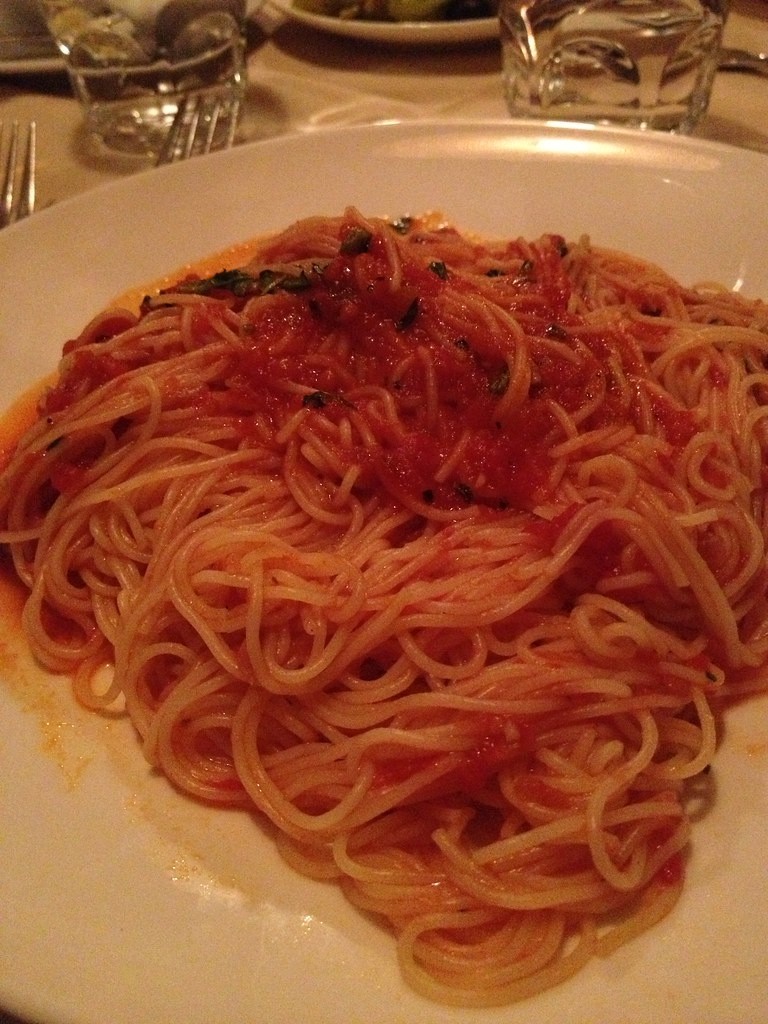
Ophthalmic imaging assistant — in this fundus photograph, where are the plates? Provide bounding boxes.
[280,0,501,45]
[2,119,767,1024]
[0,2,73,72]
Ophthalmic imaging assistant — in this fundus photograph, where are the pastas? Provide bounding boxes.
[0,208,768,1007]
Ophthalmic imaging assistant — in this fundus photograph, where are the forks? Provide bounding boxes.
[1,117,37,227]
[156,92,239,169]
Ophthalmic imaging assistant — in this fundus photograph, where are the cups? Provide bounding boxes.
[501,1,731,135]
[35,0,245,160]
